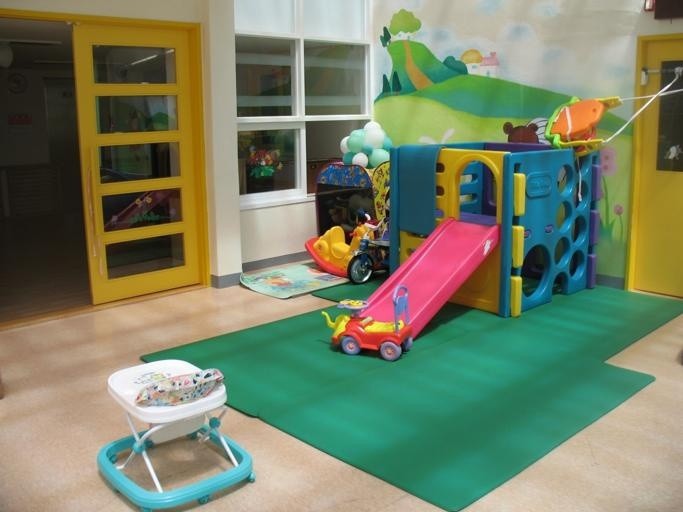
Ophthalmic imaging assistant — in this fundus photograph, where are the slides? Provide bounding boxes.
[353,215,500,343]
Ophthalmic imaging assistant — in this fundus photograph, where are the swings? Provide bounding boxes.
[547,66,683,161]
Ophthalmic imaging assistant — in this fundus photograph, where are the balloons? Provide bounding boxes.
[340,121,393,169]
